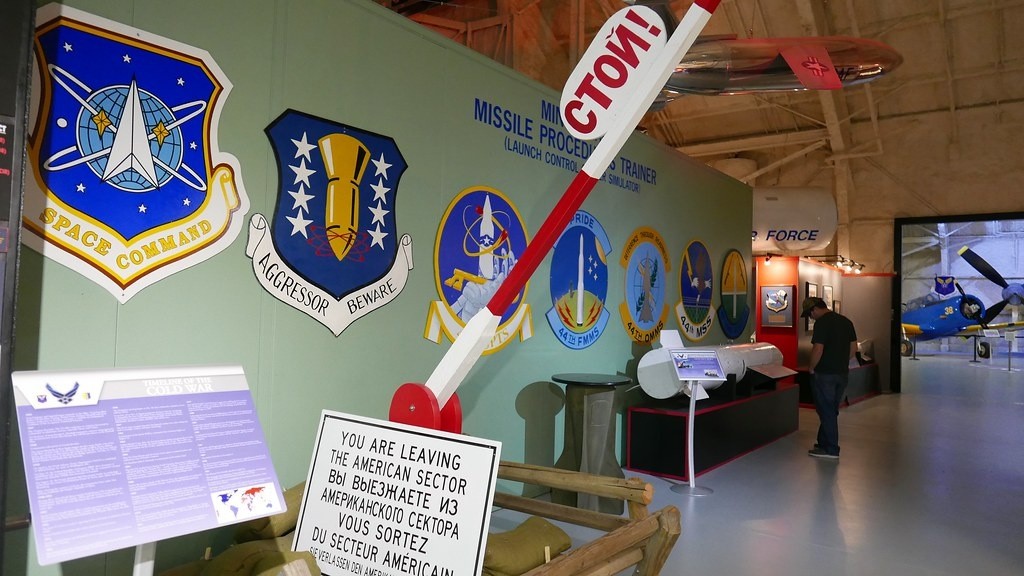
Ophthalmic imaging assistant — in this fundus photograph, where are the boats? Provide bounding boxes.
[651,34,905,110]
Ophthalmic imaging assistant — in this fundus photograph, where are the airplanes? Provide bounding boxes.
[900,280,994,359]
[678,360,692,368]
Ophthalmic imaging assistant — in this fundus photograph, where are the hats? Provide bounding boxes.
[800,297,823,318]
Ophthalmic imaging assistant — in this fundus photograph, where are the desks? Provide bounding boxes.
[551,374,633,515]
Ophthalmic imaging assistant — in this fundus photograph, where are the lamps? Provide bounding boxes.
[764,252,783,265]
[856,264,865,274]
[817,259,855,271]
[804,255,844,266]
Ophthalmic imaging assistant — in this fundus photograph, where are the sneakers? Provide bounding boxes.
[808,444,839,458]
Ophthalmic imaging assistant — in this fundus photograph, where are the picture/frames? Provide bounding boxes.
[823,285,833,311]
[834,300,841,313]
[759,284,796,328]
[805,281,818,331]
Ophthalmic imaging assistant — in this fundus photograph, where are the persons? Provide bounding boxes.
[799,296,859,459]
